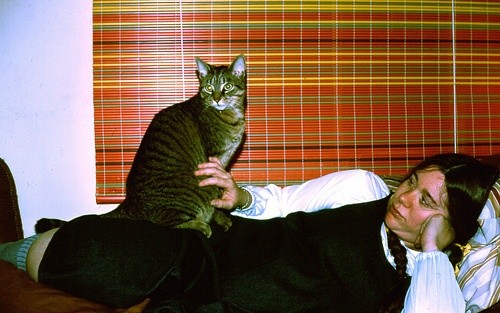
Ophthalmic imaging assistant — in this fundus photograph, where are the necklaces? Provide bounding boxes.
[237,188,250,210]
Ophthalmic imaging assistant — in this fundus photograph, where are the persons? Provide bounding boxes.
[0,152,500,313]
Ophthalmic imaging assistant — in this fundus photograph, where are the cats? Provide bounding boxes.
[35,53,248,239]
